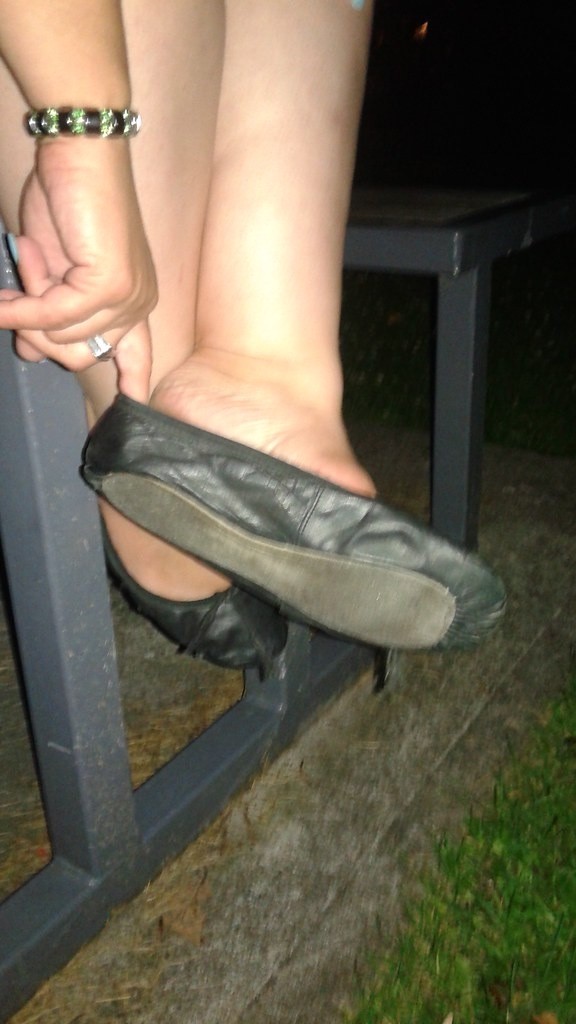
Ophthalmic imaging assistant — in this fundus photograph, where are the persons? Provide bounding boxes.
[0,3,511,671]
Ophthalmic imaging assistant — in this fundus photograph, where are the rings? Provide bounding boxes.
[88,337,117,362]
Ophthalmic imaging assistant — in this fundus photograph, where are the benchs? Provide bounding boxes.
[344,178,575,560]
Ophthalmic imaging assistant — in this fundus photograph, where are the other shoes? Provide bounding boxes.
[98,501,291,670]
[78,391,509,655]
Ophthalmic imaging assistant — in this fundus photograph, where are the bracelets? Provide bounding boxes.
[25,106,143,138]
